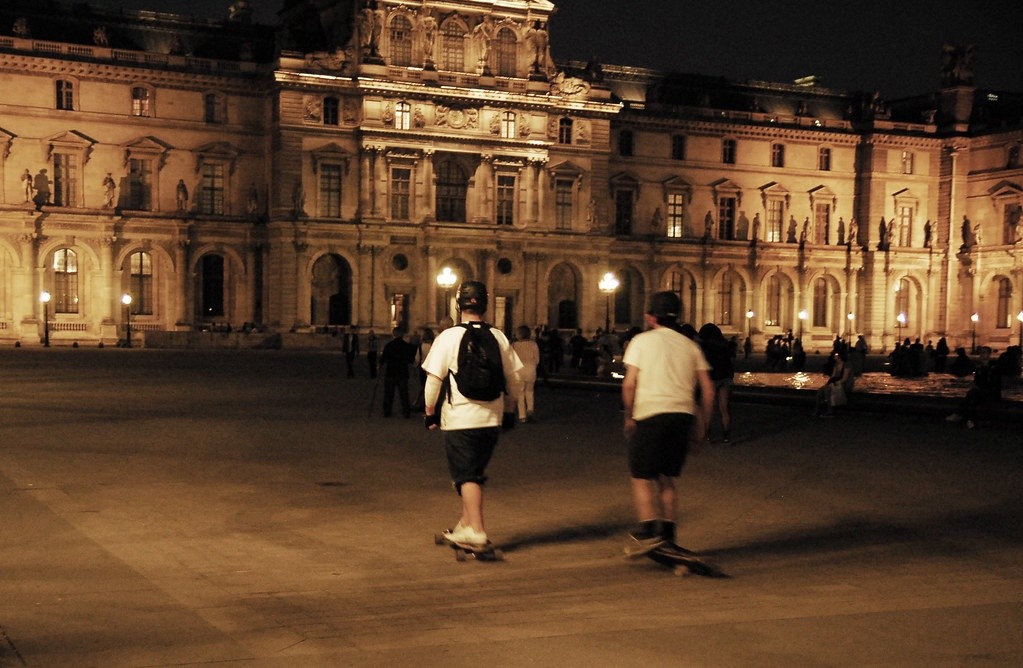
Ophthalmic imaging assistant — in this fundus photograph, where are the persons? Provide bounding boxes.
[972,222,982,245]
[817,333,869,419]
[477,15,491,59]
[885,216,895,239]
[801,216,811,240]
[20,168,33,202]
[419,279,525,545]
[766,335,808,370]
[373,3,385,50]
[421,15,439,63]
[651,207,662,231]
[927,220,936,243]
[176,179,189,210]
[378,326,438,420]
[889,332,1002,428]
[704,210,714,239]
[198,321,341,337]
[753,213,761,240]
[848,217,859,244]
[619,292,754,549]
[247,182,258,216]
[509,325,618,426]
[103,173,116,208]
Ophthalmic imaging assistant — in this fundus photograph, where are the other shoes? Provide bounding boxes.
[445,526,486,547]
[946,413,963,421]
[627,535,676,560]
[966,420,975,429]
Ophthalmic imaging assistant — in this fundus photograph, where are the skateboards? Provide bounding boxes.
[628,529,708,577]
[433,526,506,562]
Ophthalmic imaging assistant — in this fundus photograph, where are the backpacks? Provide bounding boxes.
[450,321,507,402]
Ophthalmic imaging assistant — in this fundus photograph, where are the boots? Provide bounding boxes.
[723,430,730,443]
[705,428,711,443]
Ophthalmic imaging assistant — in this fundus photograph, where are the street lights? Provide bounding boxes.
[897,315,905,345]
[746,310,753,341]
[971,313,978,352]
[598,270,621,335]
[1017,311,1023,347]
[42,293,49,347]
[799,312,806,341]
[847,311,854,345]
[123,295,132,347]
[437,268,456,321]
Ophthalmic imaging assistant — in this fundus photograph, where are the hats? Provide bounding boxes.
[461,281,488,306]
